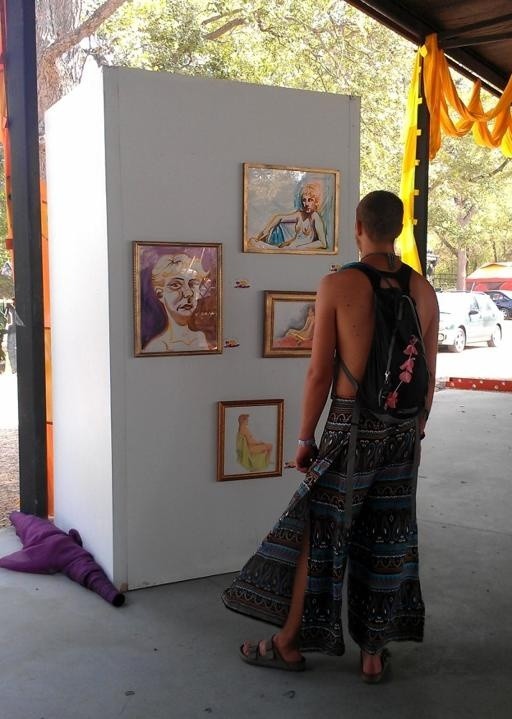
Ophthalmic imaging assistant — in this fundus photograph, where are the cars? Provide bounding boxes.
[435,288,505,354]
[483,288,512,320]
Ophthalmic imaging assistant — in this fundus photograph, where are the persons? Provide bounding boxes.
[254,179,329,250]
[143,252,210,352]
[221,191,440,685]
[285,305,315,341]
[238,413,274,467]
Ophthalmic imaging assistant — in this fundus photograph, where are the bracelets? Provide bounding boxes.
[296,438,317,446]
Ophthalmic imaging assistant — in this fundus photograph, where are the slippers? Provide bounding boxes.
[360,647,391,683]
[237,634,306,671]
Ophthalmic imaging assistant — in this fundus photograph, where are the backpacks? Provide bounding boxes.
[336,263,430,424]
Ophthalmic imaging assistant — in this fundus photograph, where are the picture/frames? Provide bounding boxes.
[243,162,339,255]
[133,240,225,358]
[217,399,286,479]
[264,290,315,358]
